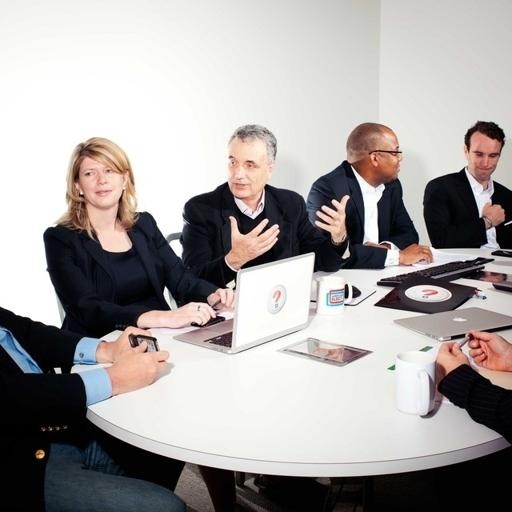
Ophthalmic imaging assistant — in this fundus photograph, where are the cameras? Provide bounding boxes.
[128,333,160,351]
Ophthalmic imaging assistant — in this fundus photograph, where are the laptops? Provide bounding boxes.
[173,250,316,353]
[393,307,512,339]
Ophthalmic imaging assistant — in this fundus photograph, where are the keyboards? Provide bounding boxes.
[378,261,486,284]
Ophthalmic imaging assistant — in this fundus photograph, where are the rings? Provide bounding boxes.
[196,305,202,311]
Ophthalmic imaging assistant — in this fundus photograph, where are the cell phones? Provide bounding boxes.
[190,315,226,327]
[491,250,512,257]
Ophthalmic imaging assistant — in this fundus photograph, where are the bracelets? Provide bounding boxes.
[330,233,349,246]
[481,215,495,229]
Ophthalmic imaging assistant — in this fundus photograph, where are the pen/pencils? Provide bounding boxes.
[458,335,470,349]
[212,300,222,310]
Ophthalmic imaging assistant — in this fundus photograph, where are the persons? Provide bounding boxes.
[45,137,239,370]
[436,330,510,445]
[423,120,511,250]
[305,122,432,271]
[1,308,189,512]
[180,124,353,291]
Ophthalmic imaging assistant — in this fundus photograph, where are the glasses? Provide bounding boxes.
[369,150,403,158]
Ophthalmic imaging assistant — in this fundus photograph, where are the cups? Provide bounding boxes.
[394,351,438,416]
[315,275,354,318]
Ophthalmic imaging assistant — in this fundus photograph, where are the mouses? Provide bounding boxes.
[345,285,362,298]
[473,257,487,264]
